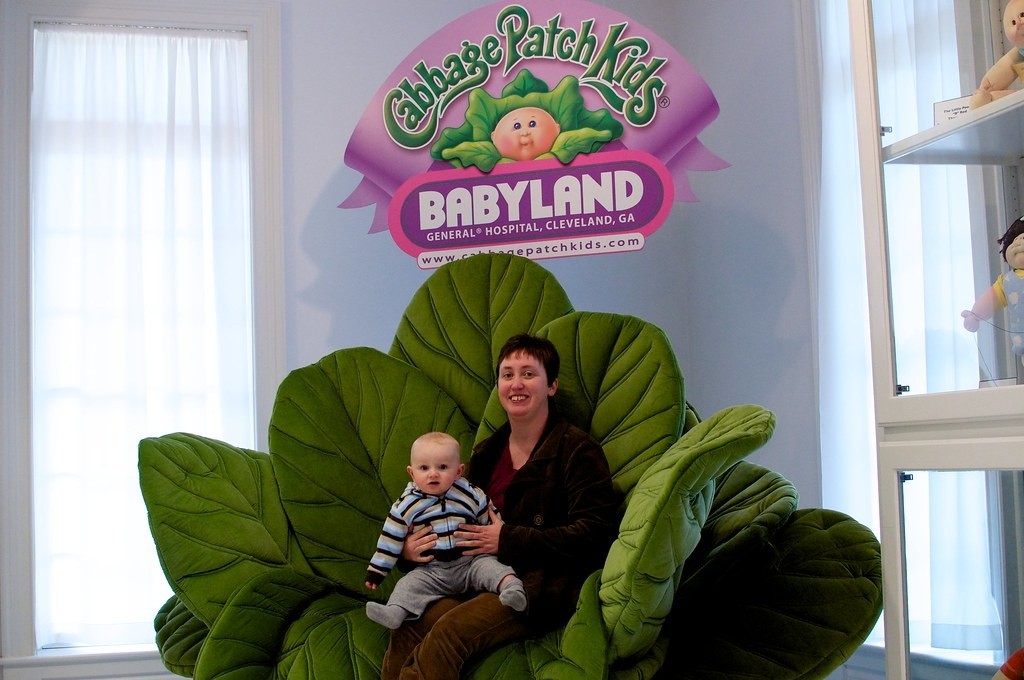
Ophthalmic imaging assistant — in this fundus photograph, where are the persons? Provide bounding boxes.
[381,333,614,680]
[365,432,527,629]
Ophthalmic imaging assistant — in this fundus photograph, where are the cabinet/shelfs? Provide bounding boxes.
[845,0,1024,680]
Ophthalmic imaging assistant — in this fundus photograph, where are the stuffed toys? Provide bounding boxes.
[961,215,1024,366]
[968,0,1024,111]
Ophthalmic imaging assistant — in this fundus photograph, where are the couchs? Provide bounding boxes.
[137,252,882,680]
